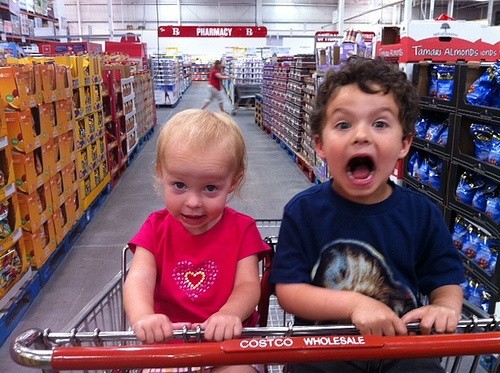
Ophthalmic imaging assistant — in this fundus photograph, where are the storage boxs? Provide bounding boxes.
[0,42,156,346]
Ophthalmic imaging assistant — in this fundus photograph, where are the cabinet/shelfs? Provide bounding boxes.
[0,2,60,42]
[150,53,215,108]
[222,35,500,373]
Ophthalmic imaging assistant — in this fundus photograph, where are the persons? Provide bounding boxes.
[269,54,468,373]
[200,60,235,115]
[124,108,272,373]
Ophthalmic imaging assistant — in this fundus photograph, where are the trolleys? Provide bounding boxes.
[222,74,262,115]
[8,216,499,368]
